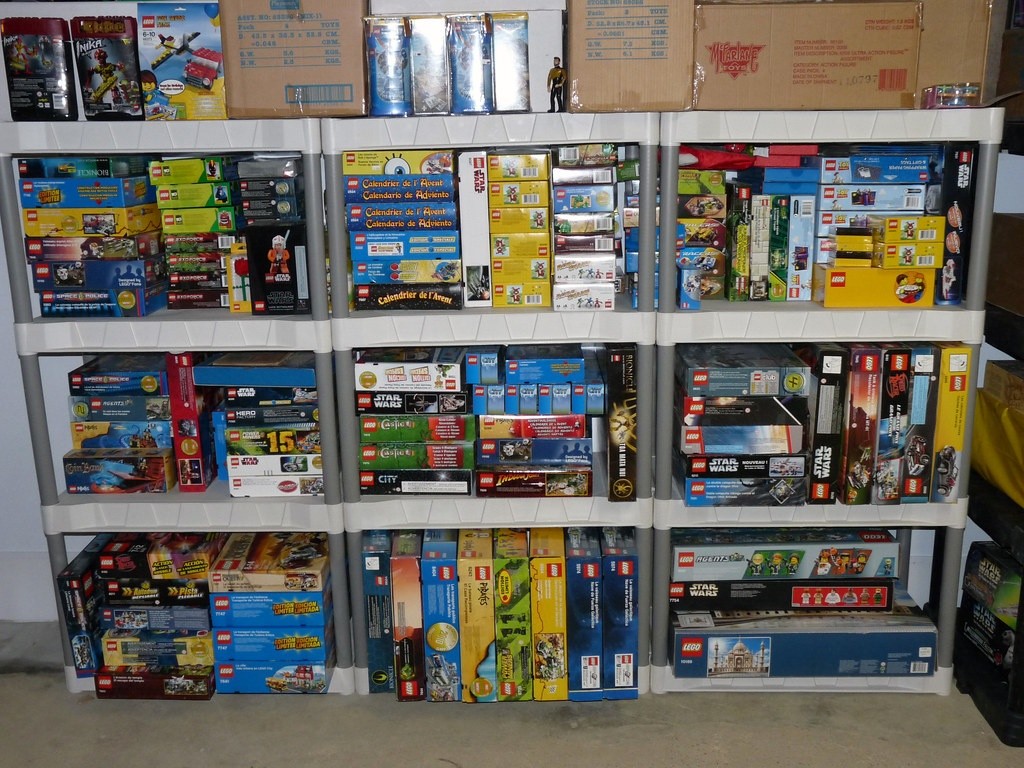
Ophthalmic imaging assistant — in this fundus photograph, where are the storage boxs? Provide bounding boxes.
[0,0,1024,703]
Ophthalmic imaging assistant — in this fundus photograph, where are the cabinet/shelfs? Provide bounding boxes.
[0,117,356,701]
[965,118,1024,580]
[651,106,1005,698]
[320,112,660,696]
